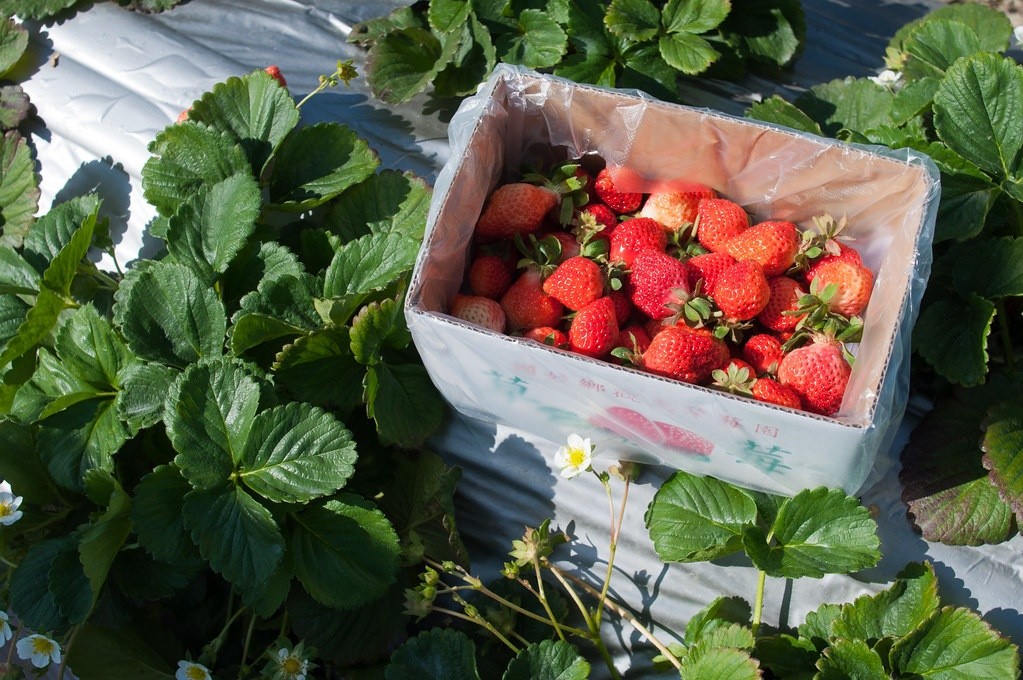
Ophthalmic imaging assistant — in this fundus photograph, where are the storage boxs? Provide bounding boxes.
[403,64,939,501]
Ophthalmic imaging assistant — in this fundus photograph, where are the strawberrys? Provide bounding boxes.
[177,106,192,123]
[265,66,287,88]
[444,164,874,419]
